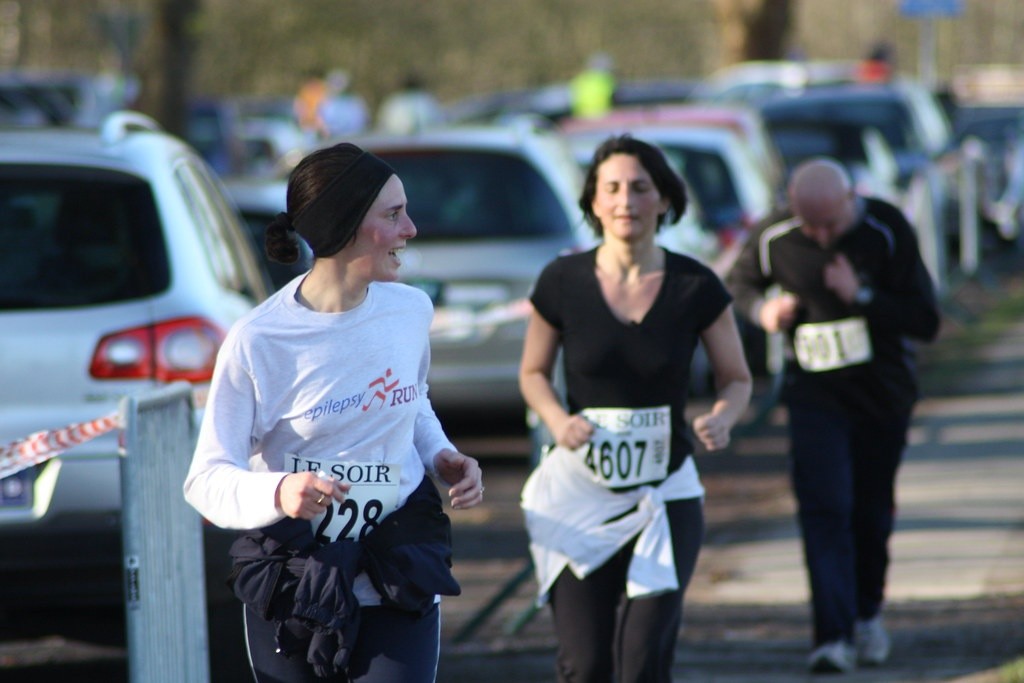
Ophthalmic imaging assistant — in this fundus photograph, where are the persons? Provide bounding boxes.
[293,62,451,140]
[517,132,754,683]
[181,142,486,682]
[723,156,940,673]
[856,36,898,86]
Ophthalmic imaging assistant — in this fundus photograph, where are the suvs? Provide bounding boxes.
[0,108,282,615]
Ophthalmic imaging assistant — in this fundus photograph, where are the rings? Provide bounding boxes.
[480,486,485,494]
[317,493,325,504]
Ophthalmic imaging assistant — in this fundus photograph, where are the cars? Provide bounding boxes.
[196,58,959,434]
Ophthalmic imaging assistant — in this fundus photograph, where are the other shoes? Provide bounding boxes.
[852,613,890,665]
[808,640,856,673]
